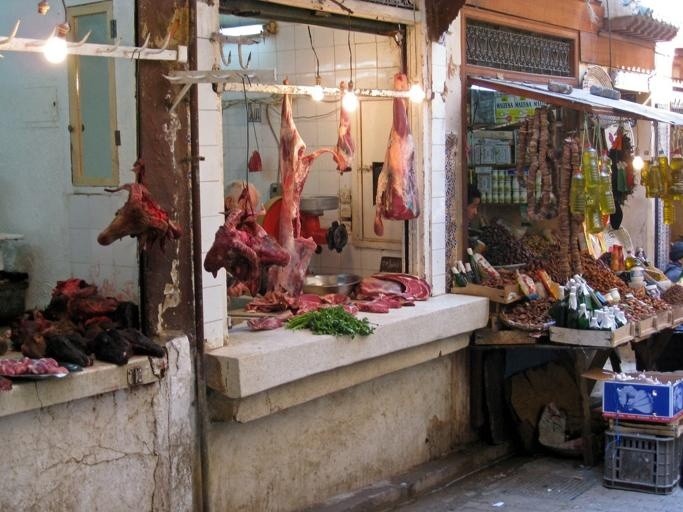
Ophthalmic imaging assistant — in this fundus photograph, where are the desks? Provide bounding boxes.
[471,328,674,465]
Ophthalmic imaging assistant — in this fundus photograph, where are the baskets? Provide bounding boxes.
[603,431,683,494]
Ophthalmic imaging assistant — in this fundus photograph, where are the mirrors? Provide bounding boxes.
[65,1,119,186]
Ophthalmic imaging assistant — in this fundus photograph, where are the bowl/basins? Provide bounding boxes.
[302,273,362,299]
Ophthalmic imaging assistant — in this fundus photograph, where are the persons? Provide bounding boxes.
[224,176,261,299]
[662,241,682,334]
[467,180,489,254]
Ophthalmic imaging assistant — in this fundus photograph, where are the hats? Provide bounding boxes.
[670,241,682,260]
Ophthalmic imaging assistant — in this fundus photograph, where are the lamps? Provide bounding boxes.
[407,2,425,103]
[41,23,69,64]
[632,119,644,172]
[220,24,267,37]
[308,25,323,101]
[343,13,359,112]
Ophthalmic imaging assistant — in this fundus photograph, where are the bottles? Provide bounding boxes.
[554,275,626,331]
[451,248,480,287]
[611,245,635,271]
[569,143,617,233]
[641,149,683,225]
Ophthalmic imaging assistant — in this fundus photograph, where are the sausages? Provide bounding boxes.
[517,106,585,286]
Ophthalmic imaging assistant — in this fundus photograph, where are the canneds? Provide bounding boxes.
[630,263,644,281]
[480,170,543,204]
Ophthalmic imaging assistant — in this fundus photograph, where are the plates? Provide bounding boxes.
[5,370,66,381]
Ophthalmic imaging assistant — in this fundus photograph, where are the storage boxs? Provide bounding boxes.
[602,370,683,418]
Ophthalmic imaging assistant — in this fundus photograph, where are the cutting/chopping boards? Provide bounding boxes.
[226,305,285,326]
